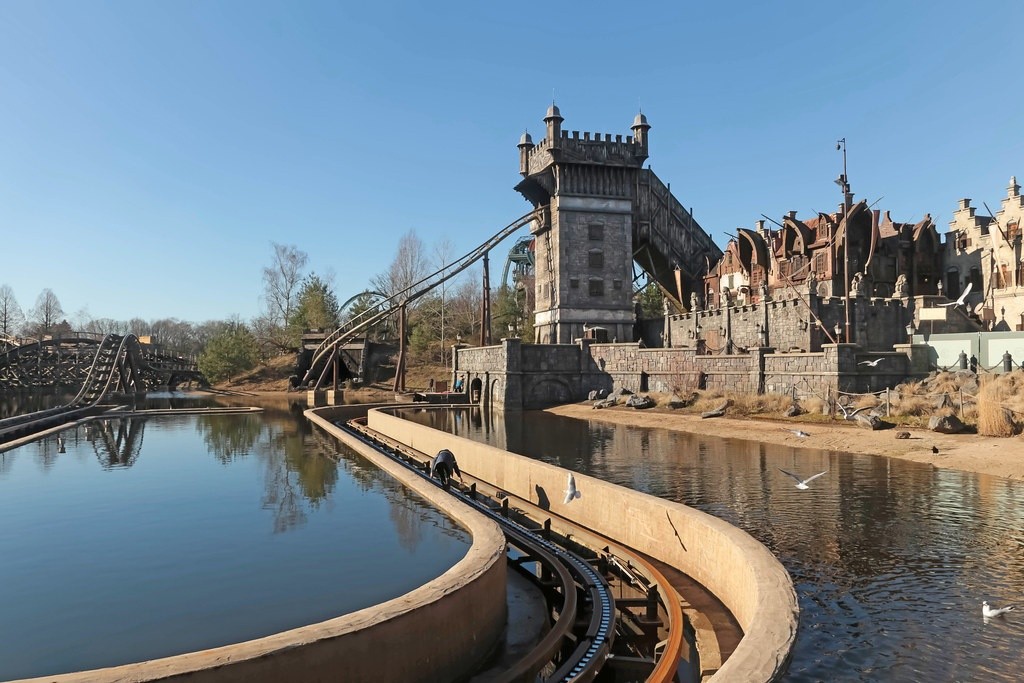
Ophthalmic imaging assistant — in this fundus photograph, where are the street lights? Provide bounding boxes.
[836,137,852,343]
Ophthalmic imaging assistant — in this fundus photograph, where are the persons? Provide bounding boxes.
[454,379,463,393]
[430,449,463,491]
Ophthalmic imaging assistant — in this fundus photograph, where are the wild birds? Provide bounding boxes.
[937,283,973,309]
[931,446,939,455]
[858,357,885,367]
[790,430,810,437]
[982,601,1015,618]
[778,468,829,490]
[836,401,875,420]
[561,472,581,505]
